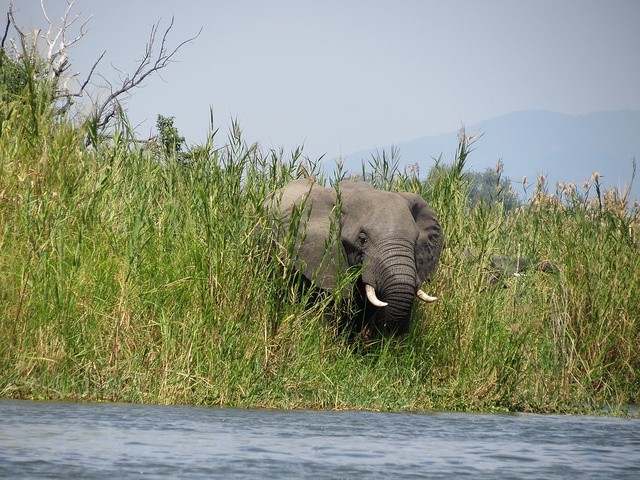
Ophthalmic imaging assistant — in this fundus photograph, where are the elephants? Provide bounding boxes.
[253,177,444,347]
